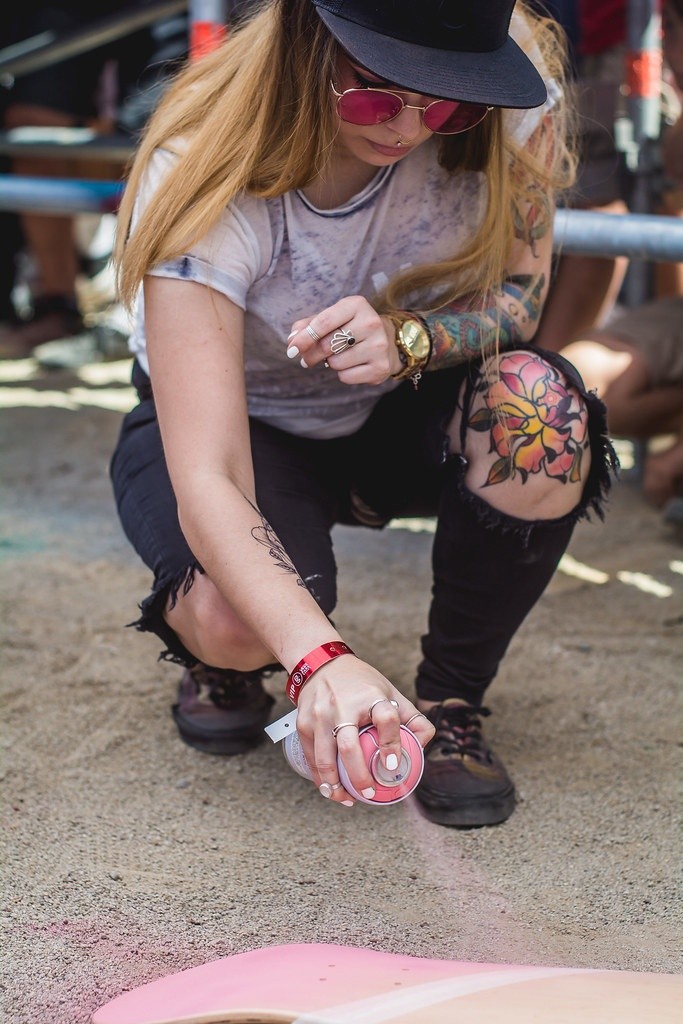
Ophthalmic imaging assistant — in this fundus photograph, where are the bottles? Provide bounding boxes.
[281,723,424,806]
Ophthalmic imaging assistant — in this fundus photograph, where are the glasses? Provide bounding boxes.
[329,76,494,135]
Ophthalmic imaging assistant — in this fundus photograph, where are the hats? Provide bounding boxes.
[312,0,548,109]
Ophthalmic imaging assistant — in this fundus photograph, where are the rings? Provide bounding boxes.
[324,358,330,368]
[404,713,427,727]
[306,325,320,342]
[367,697,399,722]
[332,723,360,738]
[329,328,356,356]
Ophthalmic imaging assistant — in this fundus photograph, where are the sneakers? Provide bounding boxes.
[168,661,275,752]
[415,698,516,825]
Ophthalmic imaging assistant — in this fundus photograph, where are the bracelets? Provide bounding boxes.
[263,640,356,744]
[405,307,432,372]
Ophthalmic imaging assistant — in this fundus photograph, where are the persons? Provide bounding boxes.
[110,0,618,827]
[1,0,682,504]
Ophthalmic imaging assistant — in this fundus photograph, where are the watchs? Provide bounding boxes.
[379,312,431,390]
